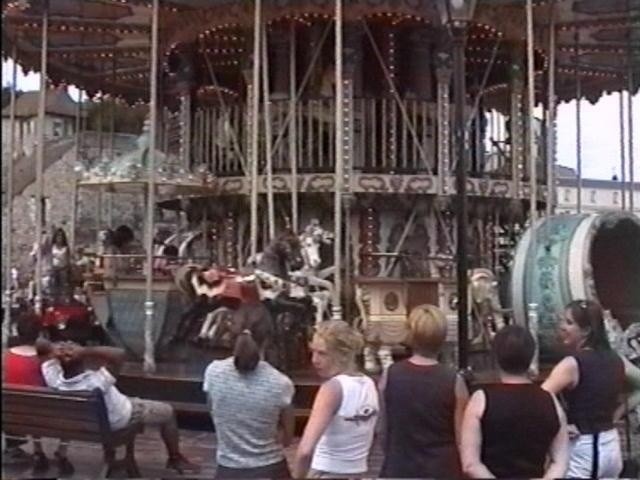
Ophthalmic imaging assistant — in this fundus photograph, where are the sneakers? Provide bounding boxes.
[165,453,200,470]
[2,447,75,473]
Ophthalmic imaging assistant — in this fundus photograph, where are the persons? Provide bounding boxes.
[540,299,639,478]
[459,324,569,480]
[101,226,134,283]
[376,302,470,479]
[35,301,202,475]
[295,318,381,479]
[201,301,295,479]
[497,251,514,327]
[51,229,72,290]
[2,309,79,478]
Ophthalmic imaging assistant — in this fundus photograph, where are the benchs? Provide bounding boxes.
[0,384,147,479]
